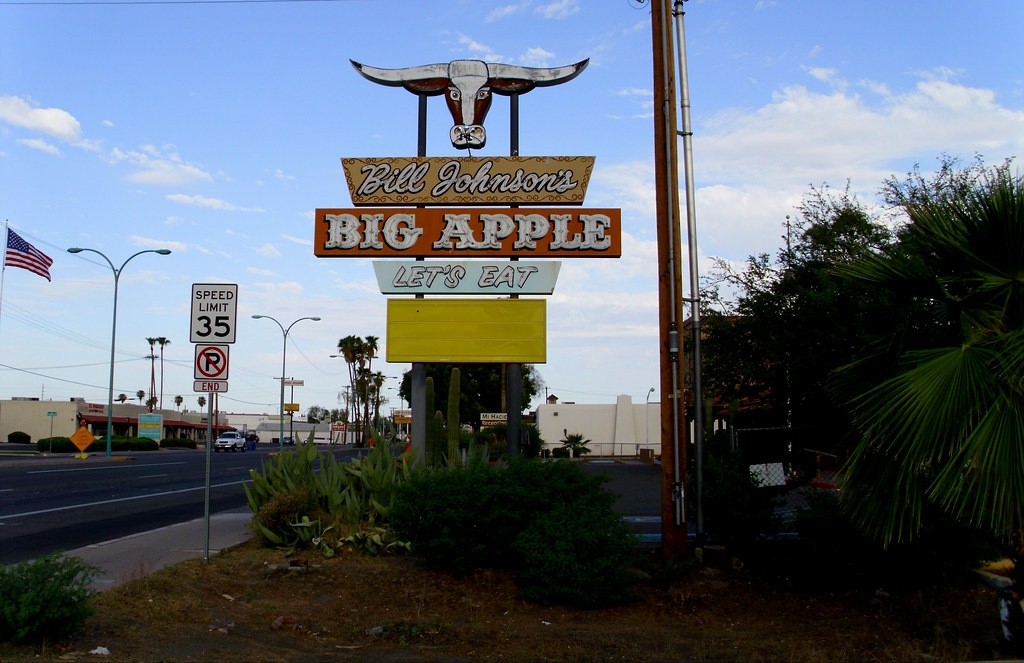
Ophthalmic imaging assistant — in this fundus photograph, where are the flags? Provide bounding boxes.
[5,227,53,282]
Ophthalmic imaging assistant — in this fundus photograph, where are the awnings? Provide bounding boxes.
[82,415,195,427]
[192,425,236,431]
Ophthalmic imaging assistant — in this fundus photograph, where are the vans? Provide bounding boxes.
[304,437,330,445]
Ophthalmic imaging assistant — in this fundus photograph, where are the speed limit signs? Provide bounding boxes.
[190,282,237,345]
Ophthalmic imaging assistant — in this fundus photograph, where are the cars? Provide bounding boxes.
[283,436,294,445]
[215,432,248,453]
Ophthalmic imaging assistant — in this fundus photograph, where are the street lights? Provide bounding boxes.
[330,355,378,449]
[646,387,655,449]
[67,247,173,457]
[387,387,404,439]
[252,314,321,454]
